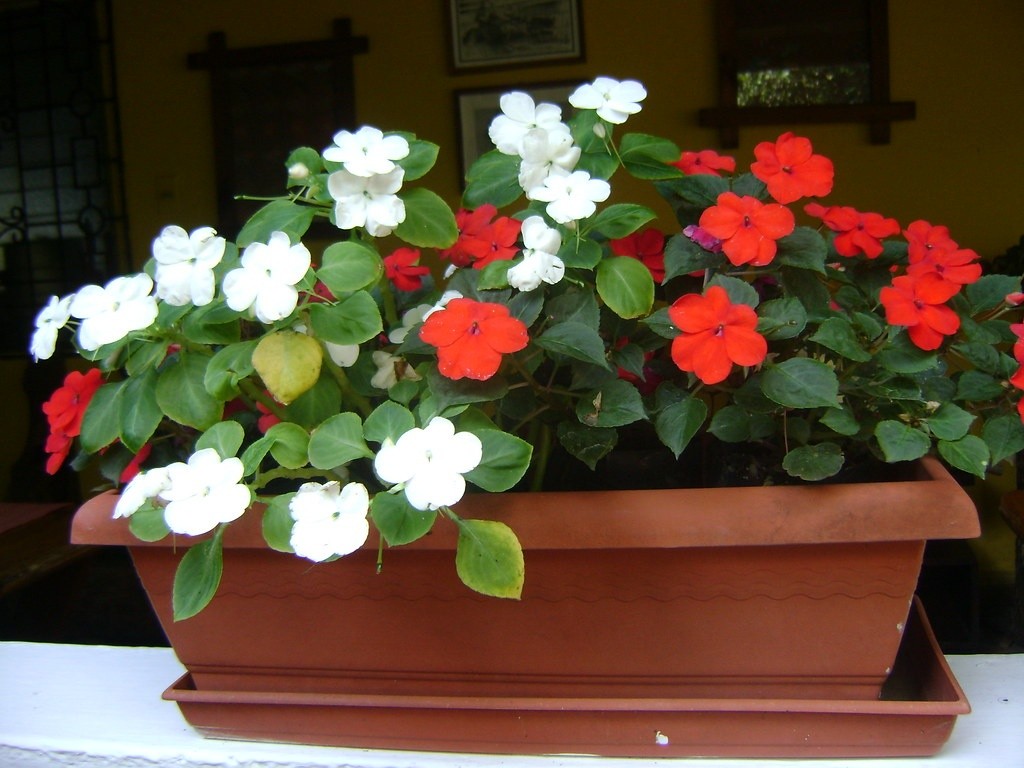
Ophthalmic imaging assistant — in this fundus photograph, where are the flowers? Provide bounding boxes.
[29,78,1024,621]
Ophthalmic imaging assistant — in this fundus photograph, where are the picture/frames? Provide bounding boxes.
[699,0,918,150]
[186,17,371,243]
[452,78,593,197]
[444,0,586,71]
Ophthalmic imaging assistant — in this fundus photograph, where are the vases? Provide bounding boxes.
[71,459,978,699]
